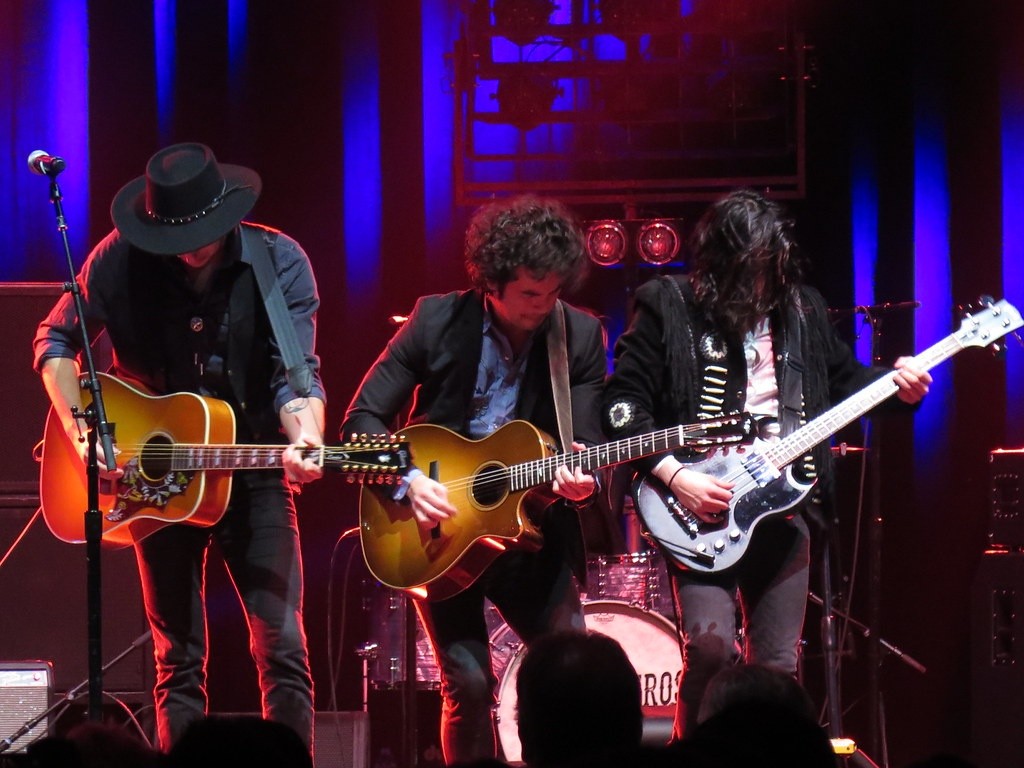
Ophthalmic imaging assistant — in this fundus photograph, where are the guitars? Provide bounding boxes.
[636,294,1024,574]
[359,410,759,604]
[38,371,415,553]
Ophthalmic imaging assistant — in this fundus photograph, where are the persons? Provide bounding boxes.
[34,141,328,755]
[24,629,838,768]
[604,189,934,745]
[339,191,609,768]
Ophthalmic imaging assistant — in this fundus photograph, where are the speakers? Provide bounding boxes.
[207,711,371,768]
[0,660,57,756]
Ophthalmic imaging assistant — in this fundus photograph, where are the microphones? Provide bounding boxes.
[28,150,65,176]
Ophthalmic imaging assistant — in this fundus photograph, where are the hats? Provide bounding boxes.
[110,143,261,255]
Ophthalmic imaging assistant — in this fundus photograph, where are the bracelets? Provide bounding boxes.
[668,466,686,489]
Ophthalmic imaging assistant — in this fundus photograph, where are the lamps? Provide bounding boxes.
[580,218,686,271]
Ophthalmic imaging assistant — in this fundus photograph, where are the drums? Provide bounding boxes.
[466,598,694,768]
[364,571,513,692]
[571,547,679,632]
